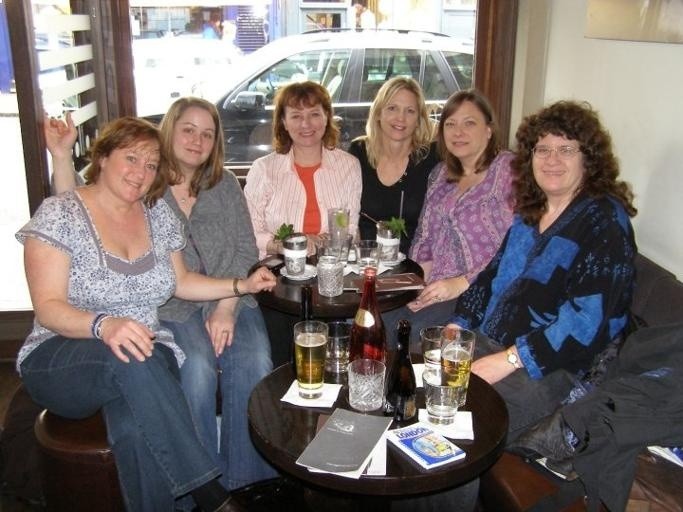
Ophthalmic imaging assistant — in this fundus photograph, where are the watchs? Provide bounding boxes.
[506,349,522,371]
[231,276,243,297]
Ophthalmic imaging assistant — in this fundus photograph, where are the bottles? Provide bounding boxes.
[383,320,416,421]
[349,268,387,376]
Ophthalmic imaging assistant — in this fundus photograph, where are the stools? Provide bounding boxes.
[36,410,113,511]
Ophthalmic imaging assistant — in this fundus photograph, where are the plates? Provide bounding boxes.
[370,251,407,267]
[279,264,316,282]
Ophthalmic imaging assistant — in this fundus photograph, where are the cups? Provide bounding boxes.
[328,207,349,240]
[419,326,444,383]
[348,359,386,411]
[283,234,306,275]
[293,321,329,400]
[325,234,352,268]
[324,322,353,373]
[439,328,476,407]
[423,367,458,425]
[356,241,382,270]
[376,222,400,265]
[316,255,344,297]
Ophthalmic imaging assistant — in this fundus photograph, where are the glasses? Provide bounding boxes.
[530,144,581,159]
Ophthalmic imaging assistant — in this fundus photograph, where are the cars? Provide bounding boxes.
[137,30,474,165]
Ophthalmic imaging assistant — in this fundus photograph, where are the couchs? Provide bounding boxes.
[486,252,683,510]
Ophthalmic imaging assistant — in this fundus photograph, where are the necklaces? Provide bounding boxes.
[172,184,193,204]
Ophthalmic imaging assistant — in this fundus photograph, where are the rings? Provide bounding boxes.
[221,329,228,334]
[432,295,444,302]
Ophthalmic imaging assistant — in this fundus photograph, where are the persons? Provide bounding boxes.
[38,94,294,511]
[383,89,521,340]
[16,118,244,512]
[390,99,639,511]
[245,81,364,256]
[348,77,446,251]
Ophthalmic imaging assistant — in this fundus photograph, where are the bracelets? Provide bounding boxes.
[90,313,113,341]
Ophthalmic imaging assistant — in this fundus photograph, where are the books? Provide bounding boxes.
[354,273,425,293]
[292,408,394,481]
[386,420,463,472]
[342,278,358,290]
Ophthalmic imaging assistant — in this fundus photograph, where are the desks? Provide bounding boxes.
[247,240,423,340]
[246,348,508,512]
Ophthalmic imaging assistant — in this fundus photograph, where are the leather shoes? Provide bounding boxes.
[188,480,234,508]
